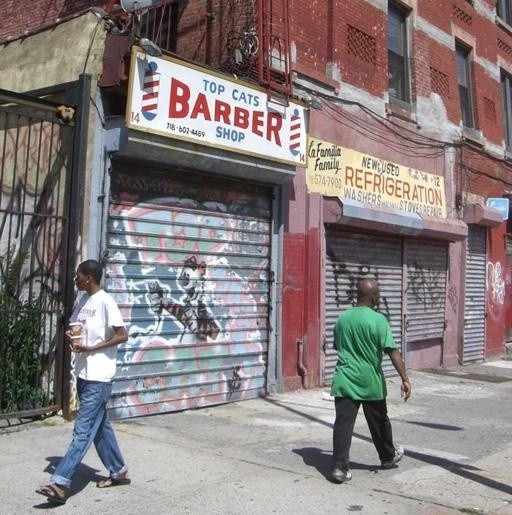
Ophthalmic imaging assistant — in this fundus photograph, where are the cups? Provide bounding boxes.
[68,321,82,336]
[71,336,83,349]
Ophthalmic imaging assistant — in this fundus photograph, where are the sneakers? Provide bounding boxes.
[381,446,405,468]
[332,468,351,482]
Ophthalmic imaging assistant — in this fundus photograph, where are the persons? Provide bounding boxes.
[34,259,131,504]
[329,278,412,483]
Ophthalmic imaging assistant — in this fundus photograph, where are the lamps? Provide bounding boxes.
[139,37,162,57]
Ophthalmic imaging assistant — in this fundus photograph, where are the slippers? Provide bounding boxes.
[97,477,130,488]
[35,484,66,504]
